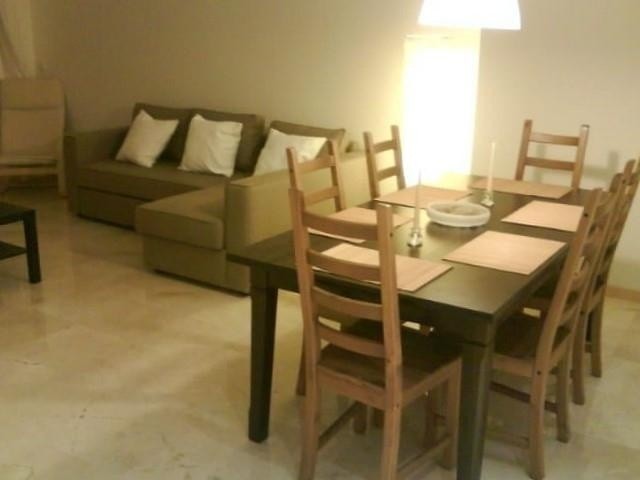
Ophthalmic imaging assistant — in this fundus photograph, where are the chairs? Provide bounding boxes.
[0,78,66,198]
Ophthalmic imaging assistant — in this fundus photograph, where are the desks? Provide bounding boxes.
[225,176,612,480]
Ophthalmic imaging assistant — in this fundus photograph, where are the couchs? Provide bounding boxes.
[67,102,370,295]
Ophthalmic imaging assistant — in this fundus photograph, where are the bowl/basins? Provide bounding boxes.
[426,200,492,227]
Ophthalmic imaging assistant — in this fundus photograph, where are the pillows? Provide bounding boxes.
[114,103,345,178]
